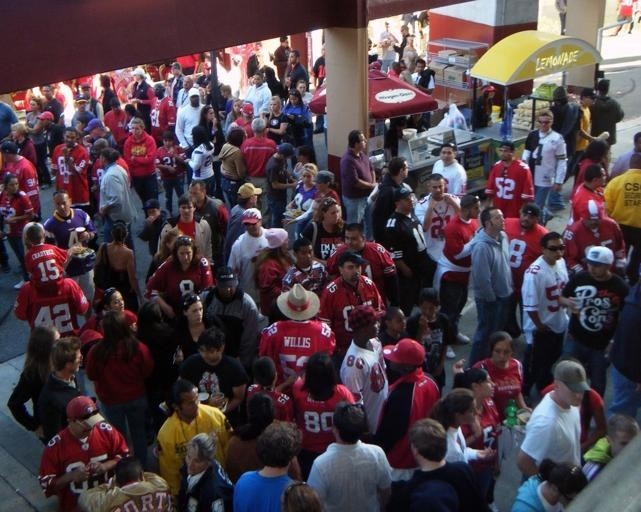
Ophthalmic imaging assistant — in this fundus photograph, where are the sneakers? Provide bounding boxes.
[447,346,456,359]
[168,211,173,218]
[14,280,25,289]
[40,184,51,189]
[457,333,470,343]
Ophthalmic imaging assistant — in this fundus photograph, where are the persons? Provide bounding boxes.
[1,21,641,512]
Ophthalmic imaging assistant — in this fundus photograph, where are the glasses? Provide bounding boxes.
[178,237,192,242]
[289,91,297,94]
[546,244,566,251]
[305,166,313,169]
[538,120,551,123]
[105,287,115,293]
[464,196,479,207]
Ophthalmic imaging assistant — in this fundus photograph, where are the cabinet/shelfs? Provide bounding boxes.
[425,36,490,91]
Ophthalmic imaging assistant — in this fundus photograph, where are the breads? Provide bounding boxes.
[511,99,551,131]
[597,131,609,142]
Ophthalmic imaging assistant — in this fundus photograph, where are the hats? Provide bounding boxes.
[383,339,425,366]
[278,144,299,157]
[540,459,588,494]
[521,203,540,215]
[131,68,144,75]
[265,228,287,248]
[83,119,103,131]
[551,87,568,100]
[500,141,515,150]
[240,103,254,113]
[582,246,614,266]
[555,360,592,392]
[36,111,54,121]
[237,183,262,198]
[216,266,238,286]
[241,208,261,224]
[394,188,414,201]
[66,396,105,427]
[76,95,88,103]
[313,171,333,182]
[0,141,20,154]
[581,88,600,98]
[339,251,369,265]
[277,284,320,320]
[189,88,200,98]
[579,199,603,218]
[142,199,160,209]
[348,306,385,328]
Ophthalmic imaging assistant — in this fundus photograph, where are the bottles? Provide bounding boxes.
[505,398,519,426]
